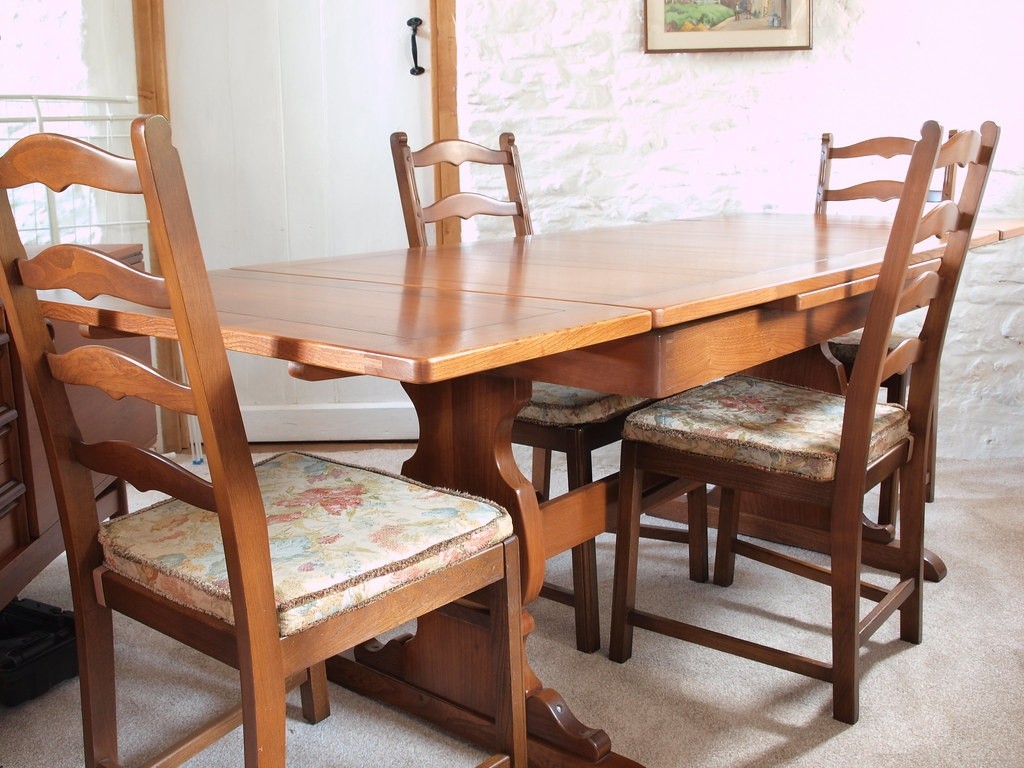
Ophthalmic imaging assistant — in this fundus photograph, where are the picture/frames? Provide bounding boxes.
[643,0,813,54]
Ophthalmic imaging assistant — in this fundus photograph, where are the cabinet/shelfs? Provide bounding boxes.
[0,245,157,609]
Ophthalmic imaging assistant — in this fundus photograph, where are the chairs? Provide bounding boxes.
[603,121,1000,725]
[811,129,963,530]
[390,131,708,653]
[0,114,529,768]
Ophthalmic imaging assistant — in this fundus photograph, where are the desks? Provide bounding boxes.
[38,213,1024,768]
